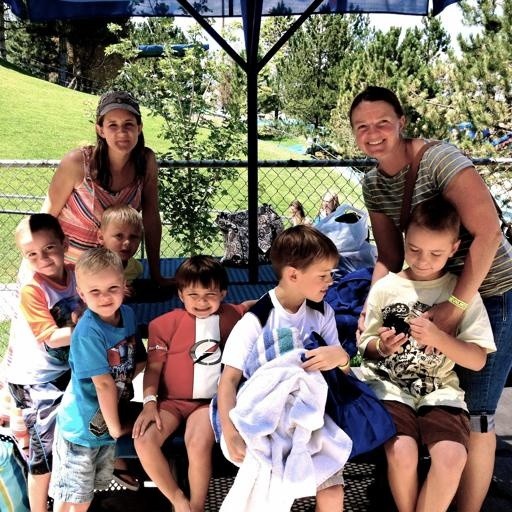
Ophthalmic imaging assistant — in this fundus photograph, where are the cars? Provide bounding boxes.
[237,113,328,143]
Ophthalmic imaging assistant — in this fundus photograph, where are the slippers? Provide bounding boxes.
[113,458,140,492]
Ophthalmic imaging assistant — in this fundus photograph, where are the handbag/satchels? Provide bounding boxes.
[327,266,376,315]
[216,202,284,268]
[315,206,377,281]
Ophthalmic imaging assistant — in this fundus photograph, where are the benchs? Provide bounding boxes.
[113,257,432,502]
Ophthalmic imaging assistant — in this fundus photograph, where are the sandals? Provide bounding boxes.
[12,435,29,458]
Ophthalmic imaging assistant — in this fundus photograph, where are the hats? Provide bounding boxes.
[96,91,141,124]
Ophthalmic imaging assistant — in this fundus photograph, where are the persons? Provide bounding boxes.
[290,201,311,226]
[2,92,162,429]
[313,192,339,225]
[48,246,147,512]
[4,212,141,512]
[94,207,145,301]
[350,203,497,511]
[348,85,512,511]
[129,255,246,512]
[208,226,358,511]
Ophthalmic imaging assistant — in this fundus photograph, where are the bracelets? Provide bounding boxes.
[142,395,157,405]
[339,354,351,368]
[447,294,473,315]
[375,338,390,360]
